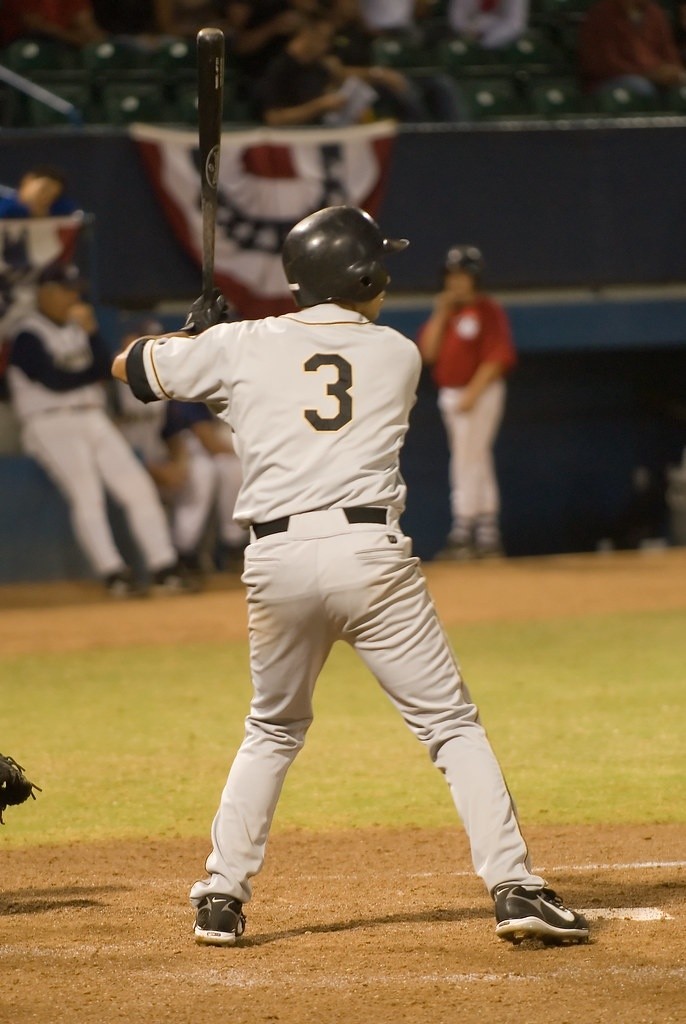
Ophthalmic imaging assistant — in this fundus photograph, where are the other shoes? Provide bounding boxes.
[103,575,149,601]
[471,542,506,559]
[434,541,467,559]
[152,572,201,595]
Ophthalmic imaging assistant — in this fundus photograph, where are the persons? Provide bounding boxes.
[110,205,592,946]
[0,162,249,600]
[0,0,531,131]
[417,245,517,561]
[576,0,686,113]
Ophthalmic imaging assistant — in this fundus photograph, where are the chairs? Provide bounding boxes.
[7,26,686,128]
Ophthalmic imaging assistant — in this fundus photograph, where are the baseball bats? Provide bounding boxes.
[195,27,226,313]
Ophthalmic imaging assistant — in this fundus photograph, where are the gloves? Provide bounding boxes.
[182,287,229,336]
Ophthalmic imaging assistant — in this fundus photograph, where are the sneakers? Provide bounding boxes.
[495,885,589,943]
[193,894,246,944]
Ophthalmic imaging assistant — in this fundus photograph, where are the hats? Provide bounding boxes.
[39,263,91,290]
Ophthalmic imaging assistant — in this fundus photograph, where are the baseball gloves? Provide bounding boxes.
[1,754,42,825]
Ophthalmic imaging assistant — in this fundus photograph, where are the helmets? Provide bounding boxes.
[281,205,410,308]
[444,245,486,276]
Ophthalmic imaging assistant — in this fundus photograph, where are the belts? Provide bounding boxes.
[253,506,387,539]
